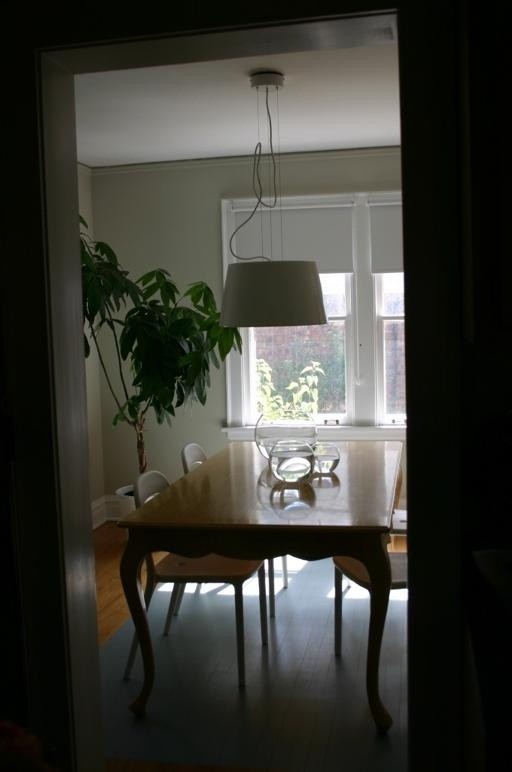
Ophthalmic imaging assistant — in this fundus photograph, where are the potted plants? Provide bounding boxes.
[81,214,242,543]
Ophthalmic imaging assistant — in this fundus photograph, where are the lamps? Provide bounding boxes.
[220,72,328,326]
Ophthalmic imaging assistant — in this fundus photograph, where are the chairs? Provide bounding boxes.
[324,549,408,669]
[122,468,275,702]
[387,443,409,543]
[177,446,293,618]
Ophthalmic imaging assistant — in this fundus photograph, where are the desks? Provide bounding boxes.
[125,435,404,701]
